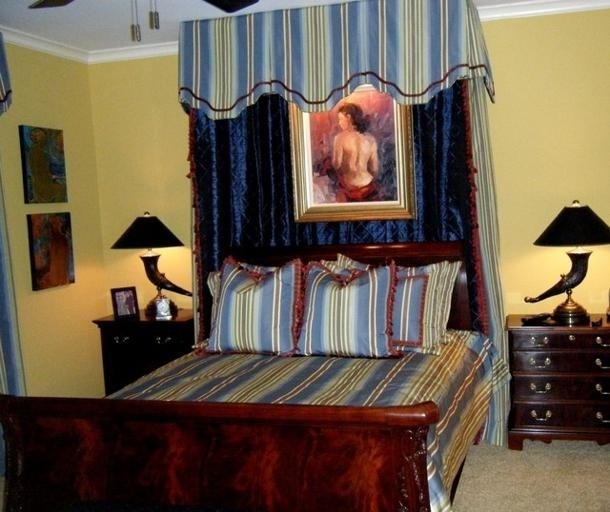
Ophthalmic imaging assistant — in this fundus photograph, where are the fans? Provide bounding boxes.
[28,0,260,13]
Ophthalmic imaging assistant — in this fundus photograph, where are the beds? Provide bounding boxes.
[0,241,514,512]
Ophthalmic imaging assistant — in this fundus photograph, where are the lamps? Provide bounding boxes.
[525,200,610,326]
[111,211,192,317]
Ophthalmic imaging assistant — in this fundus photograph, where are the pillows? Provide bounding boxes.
[192,253,463,358]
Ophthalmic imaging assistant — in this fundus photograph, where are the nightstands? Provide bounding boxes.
[92,308,195,396]
[504,313,610,451]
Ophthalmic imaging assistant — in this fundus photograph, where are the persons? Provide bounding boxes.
[323,103,382,204]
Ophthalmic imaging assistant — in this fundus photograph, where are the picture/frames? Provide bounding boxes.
[111,286,139,320]
[289,84,418,223]
